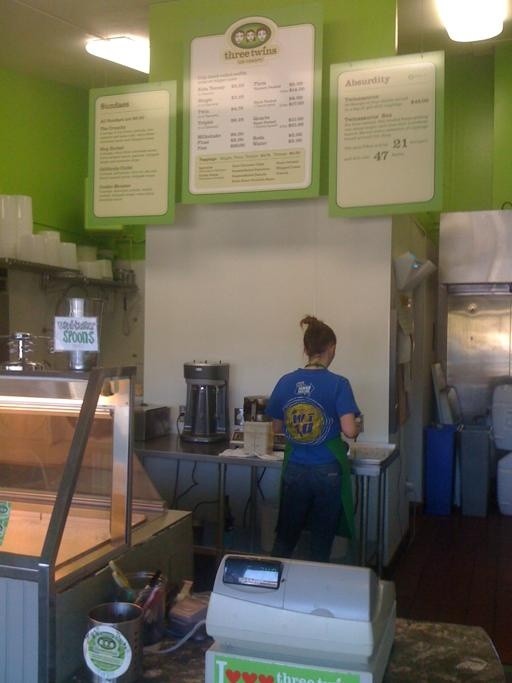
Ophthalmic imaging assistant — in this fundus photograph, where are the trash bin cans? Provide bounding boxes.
[423,422,489,518]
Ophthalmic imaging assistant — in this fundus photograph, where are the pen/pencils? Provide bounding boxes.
[108,560,167,641]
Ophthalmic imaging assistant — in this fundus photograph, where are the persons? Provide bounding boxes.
[263,316,364,561]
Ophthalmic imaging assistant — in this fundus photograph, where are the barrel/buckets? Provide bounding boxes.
[492,384,512,451]
[497,452,512,516]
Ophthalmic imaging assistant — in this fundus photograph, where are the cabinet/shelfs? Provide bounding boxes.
[0,364,193,683]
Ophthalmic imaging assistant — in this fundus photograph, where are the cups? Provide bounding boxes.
[112,571,166,646]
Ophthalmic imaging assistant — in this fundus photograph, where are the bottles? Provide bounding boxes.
[244,396,269,422]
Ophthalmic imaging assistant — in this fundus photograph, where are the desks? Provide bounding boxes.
[64,590,502,682]
[130,436,387,577]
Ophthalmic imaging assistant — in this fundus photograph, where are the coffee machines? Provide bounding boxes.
[180,360,229,443]
